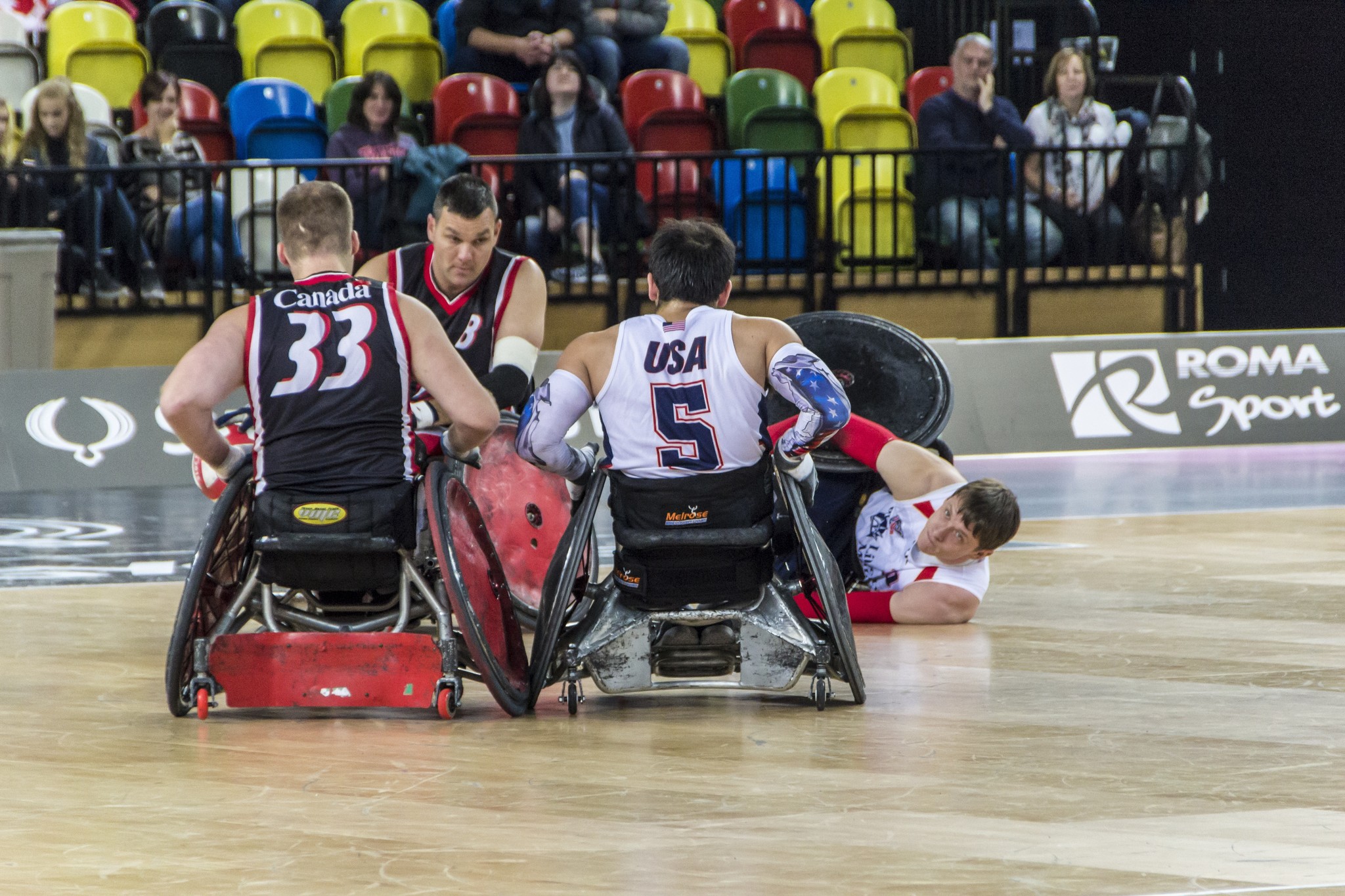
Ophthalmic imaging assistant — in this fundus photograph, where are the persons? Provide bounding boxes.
[0,0,1139,624]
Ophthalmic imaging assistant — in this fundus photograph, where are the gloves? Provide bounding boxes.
[214,443,253,483]
[213,404,253,434]
[565,442,600,516]
[440,430,482,470]
[774,437,819,509]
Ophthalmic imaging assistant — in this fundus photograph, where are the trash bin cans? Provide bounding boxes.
[0,226,64,369]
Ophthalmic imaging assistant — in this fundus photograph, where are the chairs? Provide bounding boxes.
[0,0,955,275]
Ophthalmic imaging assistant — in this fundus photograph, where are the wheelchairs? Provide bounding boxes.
[163,404,536,721]
[415,408,599,633]
[529,425,868,713]
[764,310,956,616]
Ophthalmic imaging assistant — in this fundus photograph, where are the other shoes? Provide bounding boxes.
[227,253,268,289]
[137,264,167,300]
[549,257,609,283]
[80,266,129,298]
